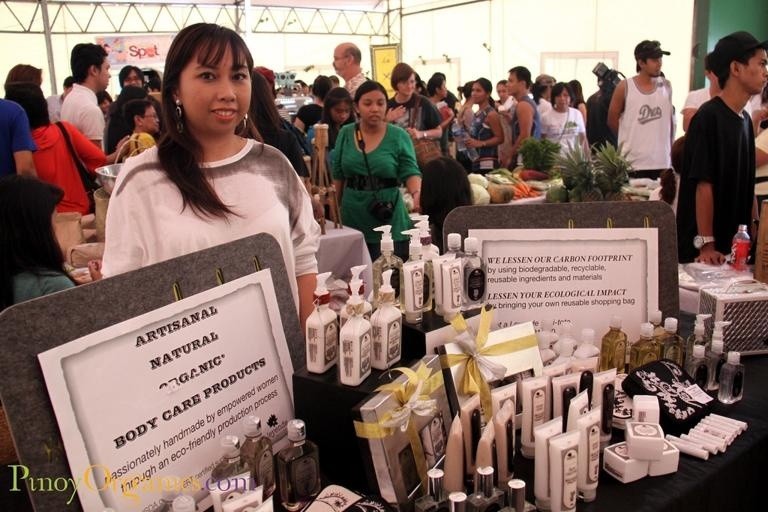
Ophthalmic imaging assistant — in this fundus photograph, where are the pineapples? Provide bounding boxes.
[549,137,637,202]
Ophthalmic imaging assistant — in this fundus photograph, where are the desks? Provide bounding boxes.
[165,312,768,512]
[489,189,549,207]
[313,217,373,304]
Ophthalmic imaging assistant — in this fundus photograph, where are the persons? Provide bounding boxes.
[0,22,768,339]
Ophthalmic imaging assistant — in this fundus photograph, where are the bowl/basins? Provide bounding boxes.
[94,162,125,196]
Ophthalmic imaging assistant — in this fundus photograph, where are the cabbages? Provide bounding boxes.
[467,173,491,205]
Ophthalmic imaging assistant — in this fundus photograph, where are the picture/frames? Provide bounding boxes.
[369,44,400,101]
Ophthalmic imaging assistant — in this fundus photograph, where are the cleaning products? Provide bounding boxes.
[177,209,748,512]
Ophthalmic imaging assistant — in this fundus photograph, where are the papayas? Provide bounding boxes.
[545,185,568,203]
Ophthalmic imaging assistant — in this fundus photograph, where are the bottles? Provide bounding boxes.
[452,117,479,162]
[730,223,750,270]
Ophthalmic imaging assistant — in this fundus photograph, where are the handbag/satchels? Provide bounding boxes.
[412,138,443,168]
[76,159,102,201]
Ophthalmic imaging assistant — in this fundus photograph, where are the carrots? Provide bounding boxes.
[508,177,542,200]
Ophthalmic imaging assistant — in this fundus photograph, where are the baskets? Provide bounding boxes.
[698,278,768,355]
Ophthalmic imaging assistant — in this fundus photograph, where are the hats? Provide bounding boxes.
[712,31,768,75]
[636,40,670,59]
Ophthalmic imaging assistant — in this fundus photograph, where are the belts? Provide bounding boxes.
[346,174,398,190]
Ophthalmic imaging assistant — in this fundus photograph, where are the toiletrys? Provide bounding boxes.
[160,219,748,512]
